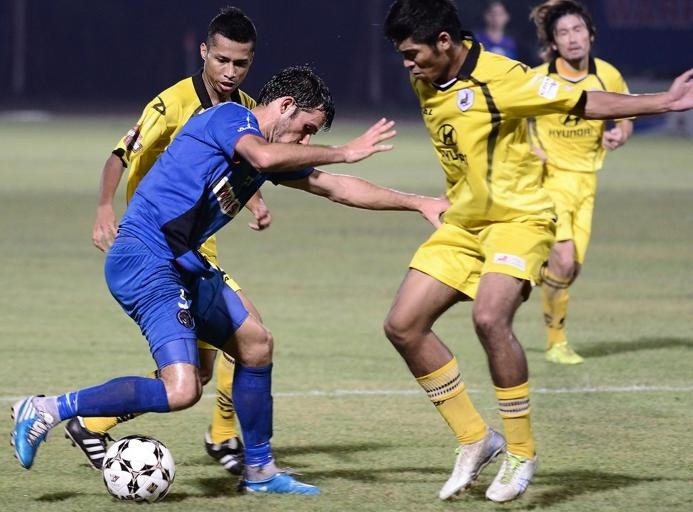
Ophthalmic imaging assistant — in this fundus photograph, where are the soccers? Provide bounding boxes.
[102,434,175,504]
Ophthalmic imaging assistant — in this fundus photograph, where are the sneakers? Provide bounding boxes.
[544,343,584,365]
[202,423,244,475]
[61,414,111,470]
[8,392,50,470]
[238,467,320,497]
[484,451,539,502]
[437,426,506,502]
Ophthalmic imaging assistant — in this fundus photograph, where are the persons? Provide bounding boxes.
[9,66,453,496]
[381,2,692,504]
[471,1,517,63]
[64,5,269,474]
[530,0,636,365]
